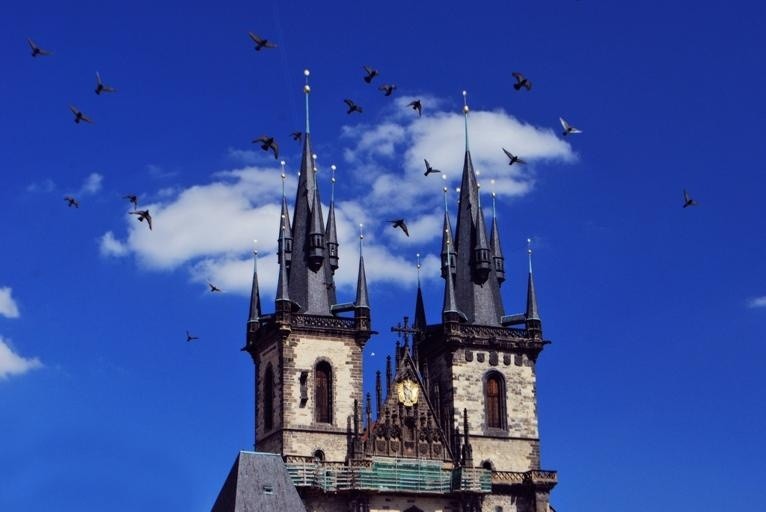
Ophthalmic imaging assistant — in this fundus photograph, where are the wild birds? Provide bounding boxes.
[501,147,529,167]
[121,193,137,211]
[510,70,533,93]
[385,217,410,238]
[128,209,152,230]
[26,35,51,59]
[342,64,423,119]
[422,158,442,177]
[208,283,221,293]
[682,189,697,209]
[94,70,116,96]
[184,329,200,342]
[63,195,79,209]
[248,32,277,52]
[68,102,93,125]
[250,135,278,161]
[559,117,583,137]
[288,130,302,145]
